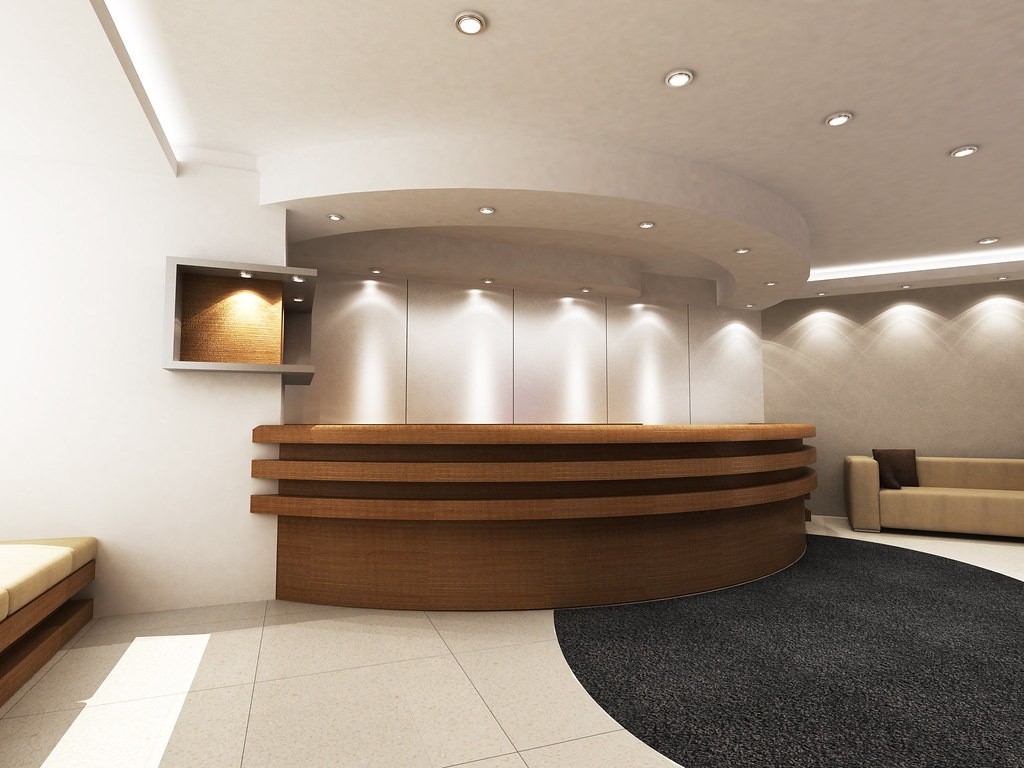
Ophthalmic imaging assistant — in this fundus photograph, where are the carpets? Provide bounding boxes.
[553,534,1024,768]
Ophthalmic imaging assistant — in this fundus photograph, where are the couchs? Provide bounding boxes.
[843,449,1024,537]
[0,537,99,710]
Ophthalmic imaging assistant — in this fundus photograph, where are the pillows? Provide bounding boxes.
[876,450,920,486]
[872,449,901,490]
